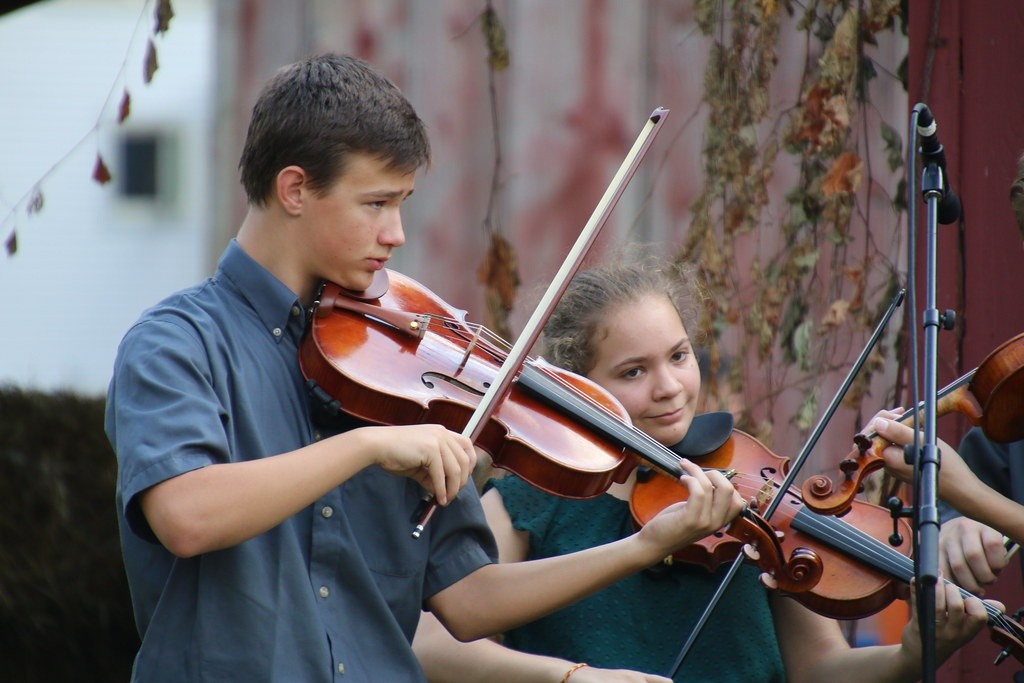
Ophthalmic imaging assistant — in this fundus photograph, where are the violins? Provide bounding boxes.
[629,413,1024,668]
[800,333,1024,518]
[296,265,826,592]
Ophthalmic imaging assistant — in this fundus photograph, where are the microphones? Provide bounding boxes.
[916,103,961,225]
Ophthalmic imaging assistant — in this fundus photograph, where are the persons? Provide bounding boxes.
[408,255,1022,683]
[860,397,1024,680]
[104,53,747,682]
